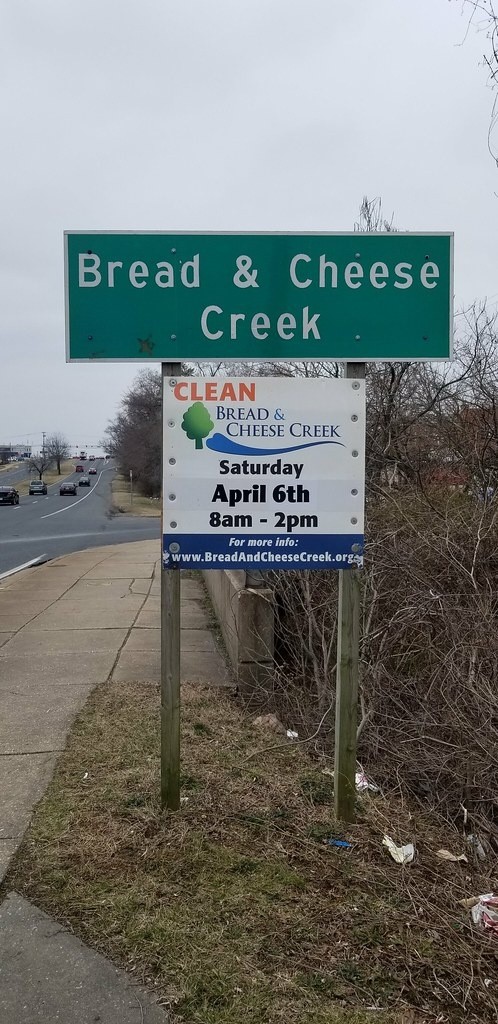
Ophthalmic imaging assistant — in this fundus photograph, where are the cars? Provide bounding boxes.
[59,482,78,496]
[0,486,19,504]
[6,456,31,462]
[89,468,97,474]
[79,456,87,460]
[89,455,94,461]
[79,477,90,486]
[76,465,84,472]
[29,480,48,495]
